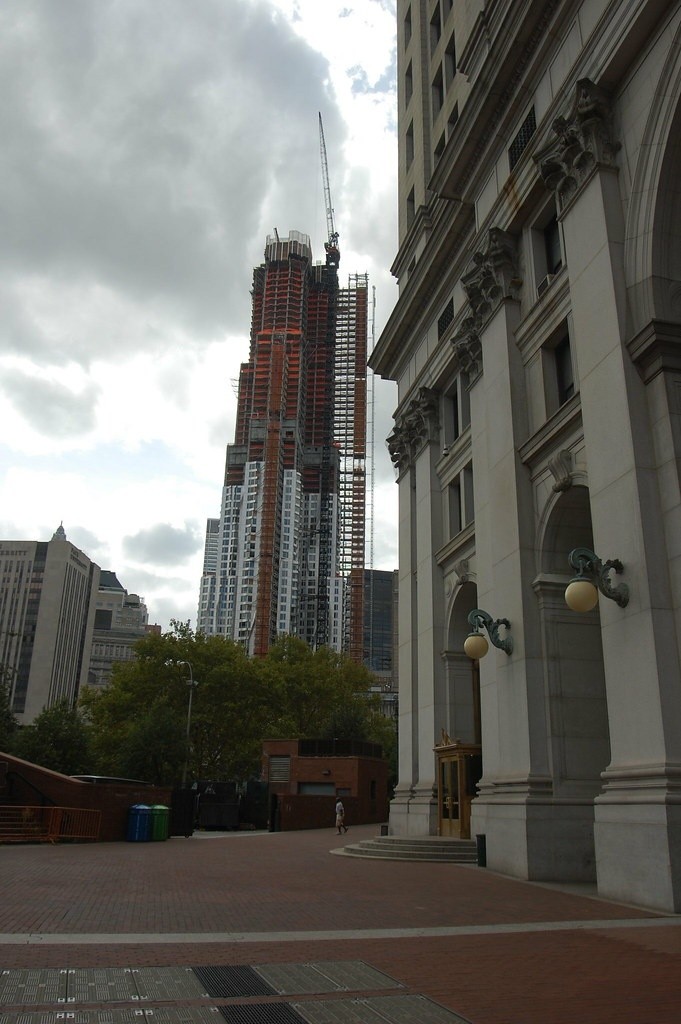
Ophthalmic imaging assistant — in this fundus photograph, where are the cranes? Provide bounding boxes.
[317,111,340,270]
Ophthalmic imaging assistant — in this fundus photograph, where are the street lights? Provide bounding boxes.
[175,659,198,789]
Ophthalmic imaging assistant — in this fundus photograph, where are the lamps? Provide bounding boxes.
[322,769,331,775]
[564,547,628,612]
[463,608,512,660]
[444,441,453,456]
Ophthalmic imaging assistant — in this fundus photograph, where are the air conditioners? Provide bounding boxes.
[536,272,555,300]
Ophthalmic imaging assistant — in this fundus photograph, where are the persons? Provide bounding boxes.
[335,796,349,835]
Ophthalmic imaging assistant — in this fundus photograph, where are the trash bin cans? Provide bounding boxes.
[150,804,172,842]
[126,804,151,842]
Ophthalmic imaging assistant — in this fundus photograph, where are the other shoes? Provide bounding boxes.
[345,829,348,833]
[337,832,342,835]
